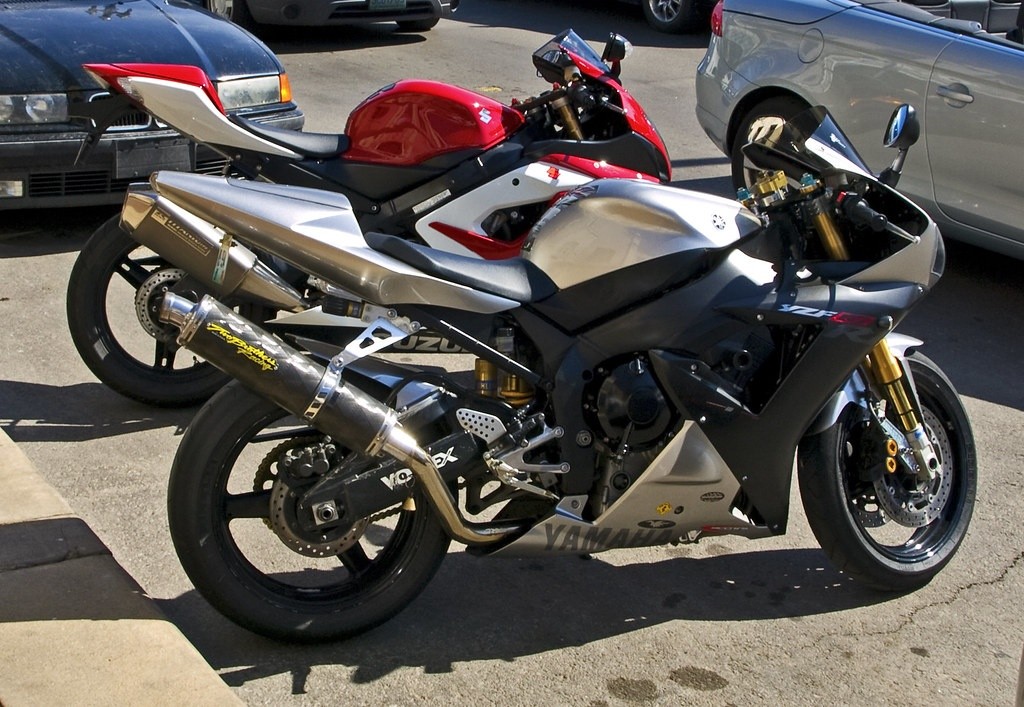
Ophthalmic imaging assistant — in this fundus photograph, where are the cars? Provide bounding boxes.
[193,1,460,33]
[0,0,305,214]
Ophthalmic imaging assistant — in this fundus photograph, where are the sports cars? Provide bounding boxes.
[691,1,1024,277]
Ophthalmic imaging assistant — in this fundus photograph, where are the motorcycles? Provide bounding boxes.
[149,100,983,645]
[66,27,674,415]
